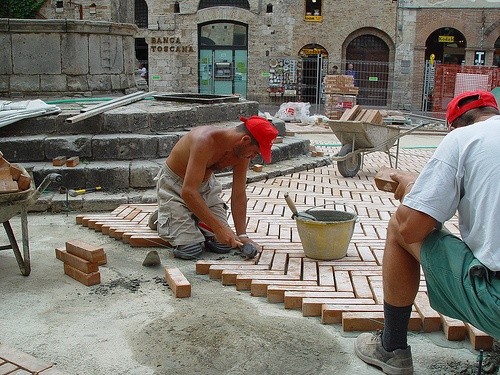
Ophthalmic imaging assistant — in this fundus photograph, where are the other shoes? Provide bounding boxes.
[148,210,158,230]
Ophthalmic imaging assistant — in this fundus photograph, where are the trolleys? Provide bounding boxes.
[327,120,430,177]
[0,168,63,277]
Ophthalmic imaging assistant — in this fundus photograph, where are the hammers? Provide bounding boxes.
[198,221,257,258]
[69,187,101,196]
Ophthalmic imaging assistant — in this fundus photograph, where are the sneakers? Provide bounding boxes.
[354,329,413,375]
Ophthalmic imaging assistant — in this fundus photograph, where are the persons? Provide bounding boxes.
[346,62,357,85]
[330,65,339,74]
[147,116,278,260]
[139,64,146,78]
[353,89,500,375]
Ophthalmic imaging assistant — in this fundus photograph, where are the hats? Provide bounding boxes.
[446,90,499,125]
[240,116,279,164]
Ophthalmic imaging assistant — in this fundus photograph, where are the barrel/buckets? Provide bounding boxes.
[294,202,360,261]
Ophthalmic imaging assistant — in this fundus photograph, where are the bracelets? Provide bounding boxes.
[404,181,415,188]
[237,235,250,237]
